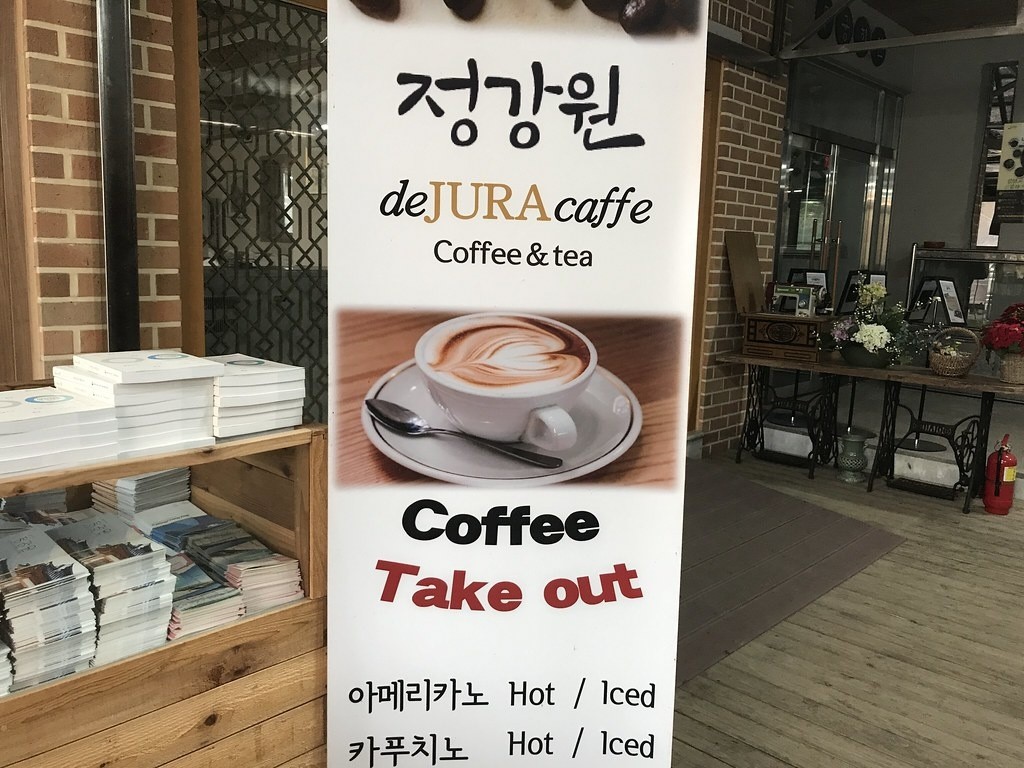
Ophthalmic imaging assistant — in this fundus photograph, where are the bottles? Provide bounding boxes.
[838,434,868,482]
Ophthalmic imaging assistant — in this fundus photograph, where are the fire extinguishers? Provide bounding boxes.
[983,433,1017,516]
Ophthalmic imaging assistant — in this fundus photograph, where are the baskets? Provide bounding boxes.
[999,352,1024,384]
[837,340,895,368]
[927,327,980,378]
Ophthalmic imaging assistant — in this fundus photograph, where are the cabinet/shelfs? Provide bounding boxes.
[0,421,329,768]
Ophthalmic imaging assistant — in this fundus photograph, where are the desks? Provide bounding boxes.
[717,351,1023,514]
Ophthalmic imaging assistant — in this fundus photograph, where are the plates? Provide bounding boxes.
[361,358,643,487]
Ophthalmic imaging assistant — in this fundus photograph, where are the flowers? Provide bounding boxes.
[832,280,1024,365]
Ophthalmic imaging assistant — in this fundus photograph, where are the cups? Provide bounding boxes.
[414,311,598,452]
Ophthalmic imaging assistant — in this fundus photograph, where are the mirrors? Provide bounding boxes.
[964,57,1024,250]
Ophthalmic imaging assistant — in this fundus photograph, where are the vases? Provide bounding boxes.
[839,339,898,369]
[999,351,1023,384]
[837,434,868,483]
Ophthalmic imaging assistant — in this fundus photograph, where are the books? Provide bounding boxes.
[0,466,305,701]
[0,349,306,479]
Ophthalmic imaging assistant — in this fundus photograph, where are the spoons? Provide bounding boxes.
[364,399,563,469]
